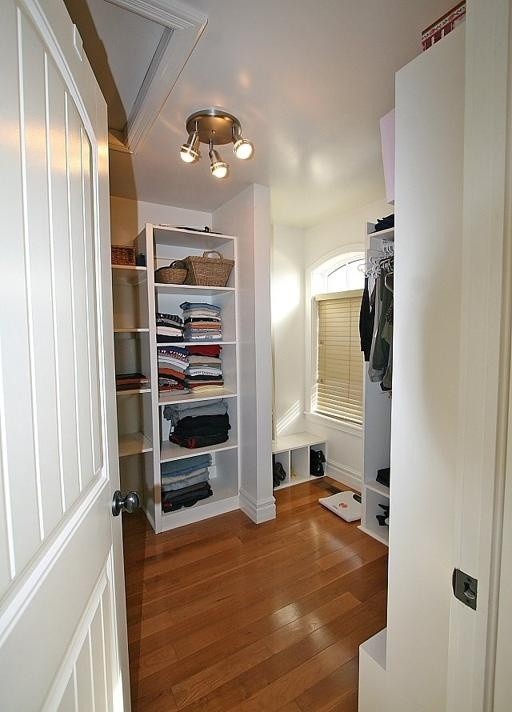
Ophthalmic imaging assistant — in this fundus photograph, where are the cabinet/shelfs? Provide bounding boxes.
[355,224,399,549]
[272,432,328,492]
[111,220,243,535]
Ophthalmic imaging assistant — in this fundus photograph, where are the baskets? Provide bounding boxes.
[155,251,234,287]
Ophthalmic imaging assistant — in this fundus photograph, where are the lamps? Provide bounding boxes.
[178,108,254,178]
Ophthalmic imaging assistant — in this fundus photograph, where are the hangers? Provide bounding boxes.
[357,238,394,292]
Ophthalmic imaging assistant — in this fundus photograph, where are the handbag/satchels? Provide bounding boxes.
[310,449,325,476]
